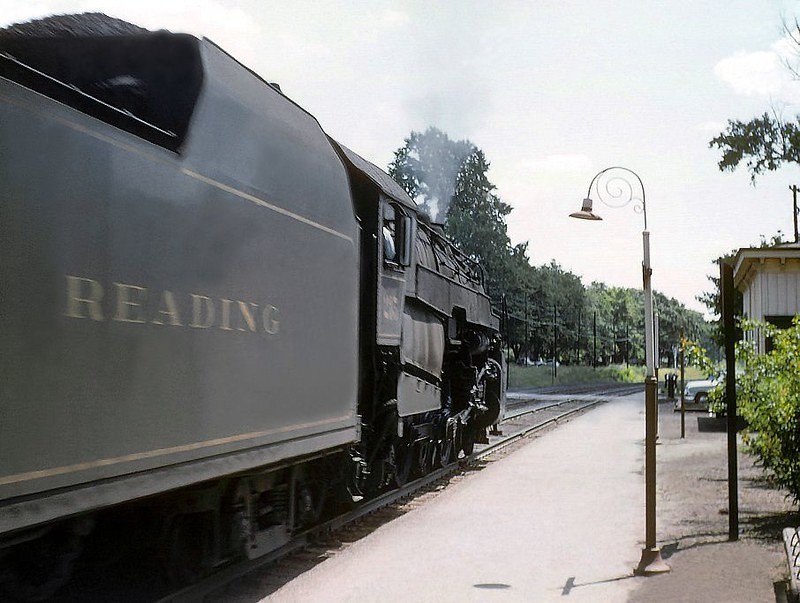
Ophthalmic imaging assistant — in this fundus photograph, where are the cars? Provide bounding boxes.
[516,357,560,367]
[684,369,763,403]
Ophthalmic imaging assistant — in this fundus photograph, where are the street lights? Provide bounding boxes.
[628,291,662,445]
[567,166,671,574]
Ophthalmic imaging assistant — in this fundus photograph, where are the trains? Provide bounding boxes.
[0,22,508,569]
